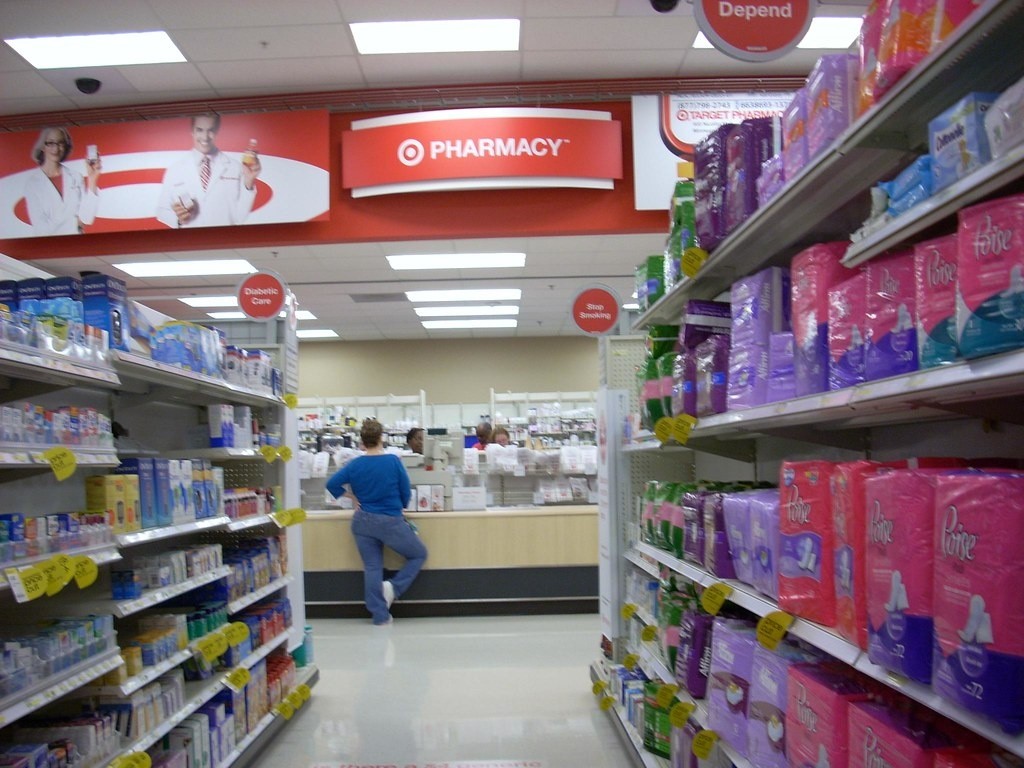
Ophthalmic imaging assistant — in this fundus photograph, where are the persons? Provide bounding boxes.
[154,111,262,228]
[25,126,103,236]
[471,422,511,449]
[326,419,428,625]
[407,428,425,454]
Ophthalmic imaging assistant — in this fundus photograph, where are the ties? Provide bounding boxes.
[200,157,210,189]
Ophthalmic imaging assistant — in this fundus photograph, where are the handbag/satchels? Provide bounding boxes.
[404,517,420,535]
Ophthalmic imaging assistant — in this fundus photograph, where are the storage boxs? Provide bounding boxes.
[0,275,315,768]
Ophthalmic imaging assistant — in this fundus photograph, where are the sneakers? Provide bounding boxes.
[383,614,393,624]
[382,581,394,609]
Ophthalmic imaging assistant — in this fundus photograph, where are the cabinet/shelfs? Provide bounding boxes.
[0,315,318,768]
[588,0,1023,768]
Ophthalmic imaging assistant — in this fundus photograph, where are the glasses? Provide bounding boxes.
[44,141,65,147]
